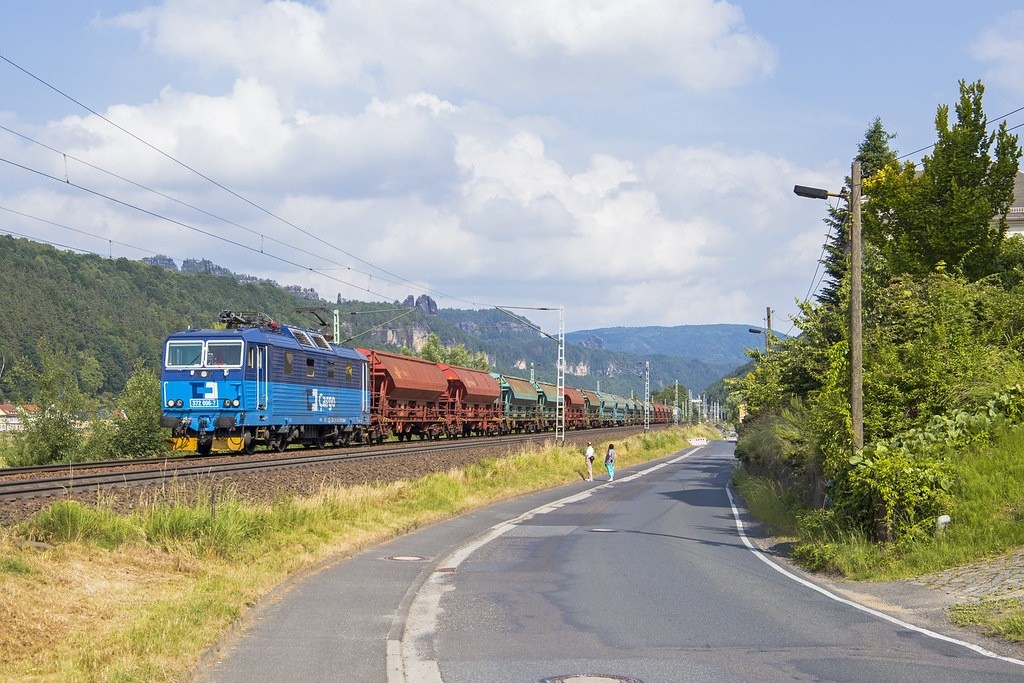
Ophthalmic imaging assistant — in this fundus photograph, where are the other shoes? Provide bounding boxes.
[607,476,613,481]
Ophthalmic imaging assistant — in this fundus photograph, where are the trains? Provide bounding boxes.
[160,309,682,454]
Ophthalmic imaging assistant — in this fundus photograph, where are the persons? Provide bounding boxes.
[585,442,594,481]
[605,444,615,482]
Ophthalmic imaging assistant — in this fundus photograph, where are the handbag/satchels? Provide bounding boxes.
[590,456,594,460]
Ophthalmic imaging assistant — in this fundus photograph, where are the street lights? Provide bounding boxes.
[793,160,864,462]
[748,328,769,360]
